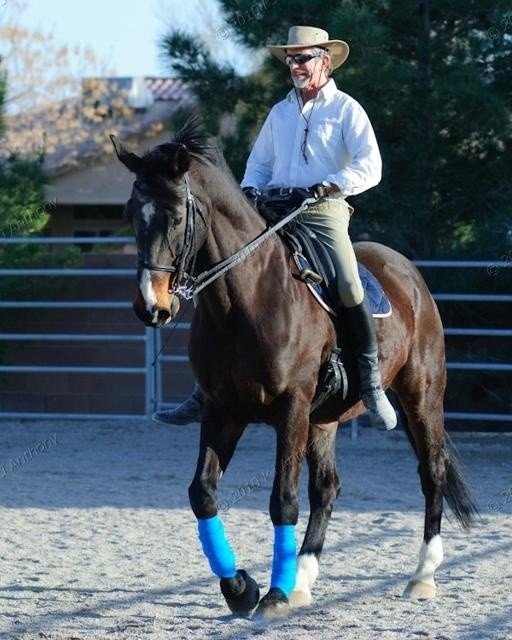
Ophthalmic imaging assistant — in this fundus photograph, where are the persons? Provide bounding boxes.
[151,24,400,434]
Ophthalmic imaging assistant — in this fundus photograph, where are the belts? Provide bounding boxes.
[266,186,306,197]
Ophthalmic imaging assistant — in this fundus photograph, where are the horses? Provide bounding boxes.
[110,110,481,622]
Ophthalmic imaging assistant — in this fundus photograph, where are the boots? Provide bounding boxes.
[152,376,205,426]
[345,291,398,432]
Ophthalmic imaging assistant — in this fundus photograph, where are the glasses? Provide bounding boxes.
[285,54,323,66]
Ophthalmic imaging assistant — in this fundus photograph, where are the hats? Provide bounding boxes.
[266,26,350,70]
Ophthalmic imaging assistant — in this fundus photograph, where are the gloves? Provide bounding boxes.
[244,186,262,205]
[288,181,332,209]
